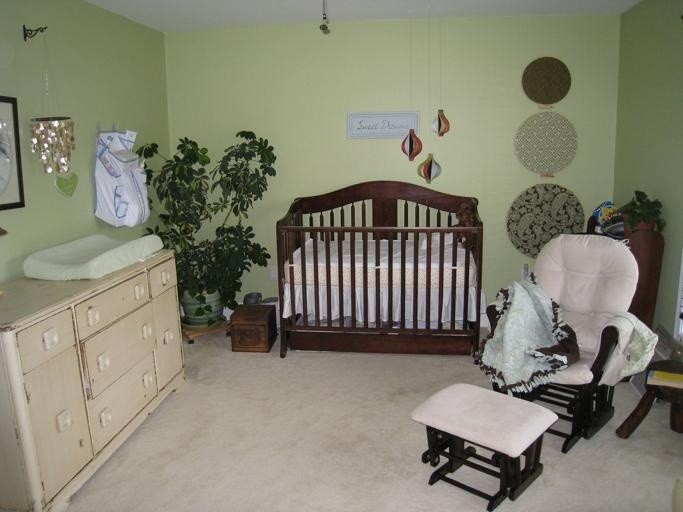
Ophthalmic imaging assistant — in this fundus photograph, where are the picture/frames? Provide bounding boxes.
[0,95,26,211]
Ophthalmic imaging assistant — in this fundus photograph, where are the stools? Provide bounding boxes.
[615,358,682,442]
[411,380,560,512]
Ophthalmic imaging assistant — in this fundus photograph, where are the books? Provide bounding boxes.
[647,370,683,389]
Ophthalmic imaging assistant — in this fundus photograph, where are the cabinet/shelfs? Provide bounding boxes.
[0,249,188,511]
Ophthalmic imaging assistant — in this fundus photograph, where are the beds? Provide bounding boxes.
[275,181,485,361]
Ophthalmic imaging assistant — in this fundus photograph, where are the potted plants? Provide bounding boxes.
[618,188,666,238]
[135,128,279,326]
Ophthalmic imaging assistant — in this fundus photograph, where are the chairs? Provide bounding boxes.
[484,233,641,456]
